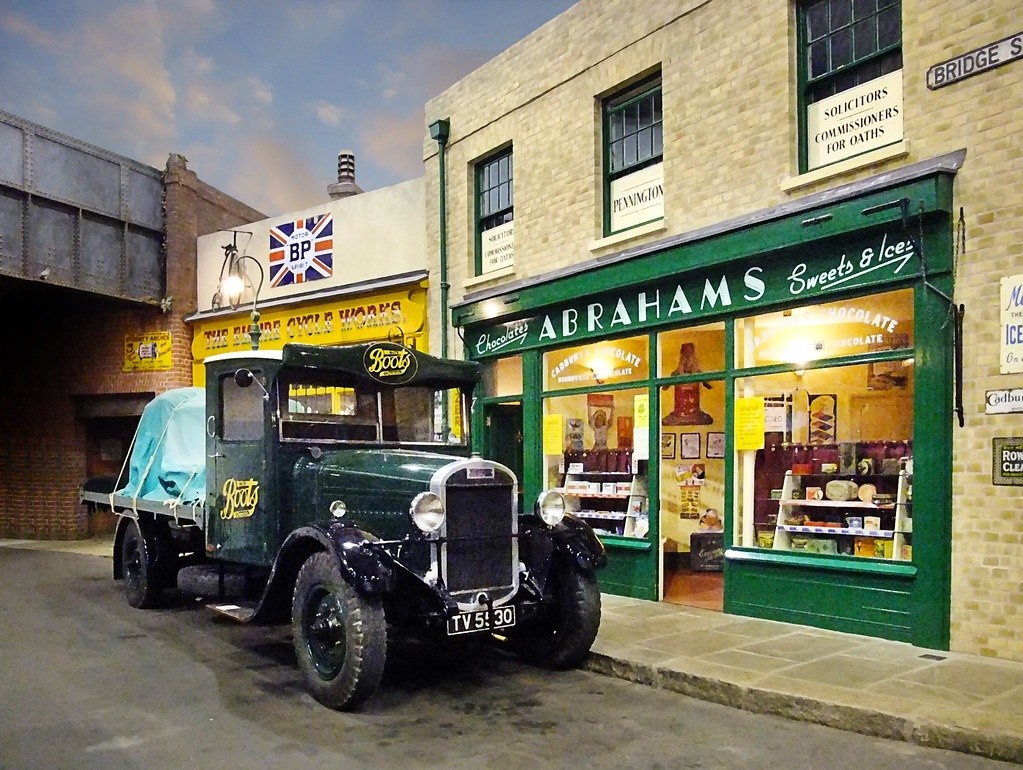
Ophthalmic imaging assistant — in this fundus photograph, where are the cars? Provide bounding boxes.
[75,326,609,713]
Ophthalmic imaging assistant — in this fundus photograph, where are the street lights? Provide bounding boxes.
[223,253,265,351]
